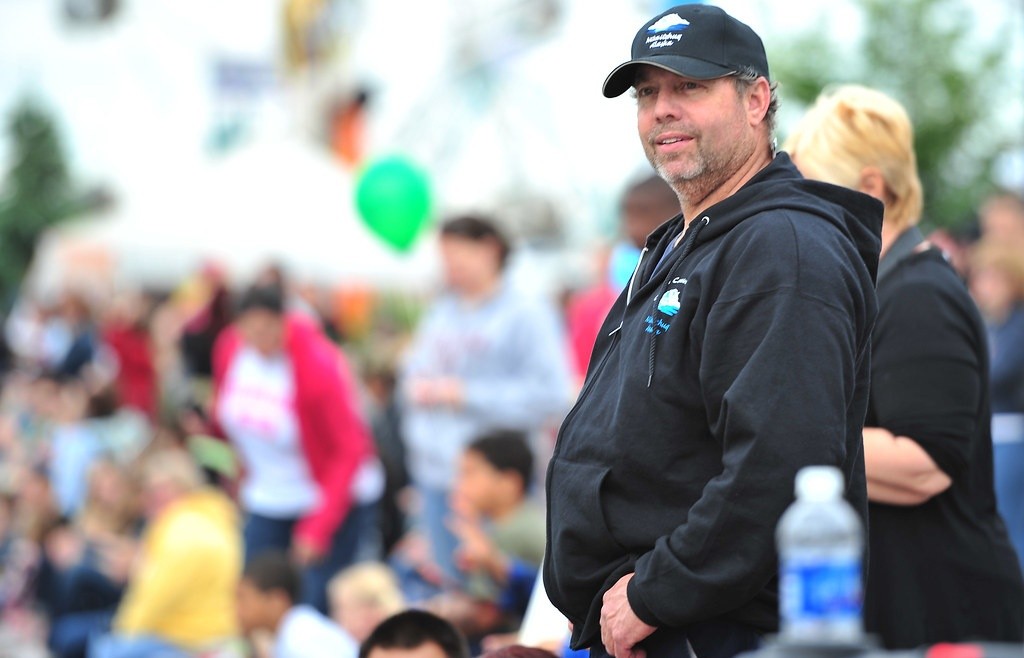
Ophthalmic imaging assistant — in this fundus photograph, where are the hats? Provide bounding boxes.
[603,4,769,98]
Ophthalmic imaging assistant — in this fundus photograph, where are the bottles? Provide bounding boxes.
[774,466,864,641]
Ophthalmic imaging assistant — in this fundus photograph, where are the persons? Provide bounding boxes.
[543,4,885,658]
[783,87,1024,649]
[0,179,682,658]
